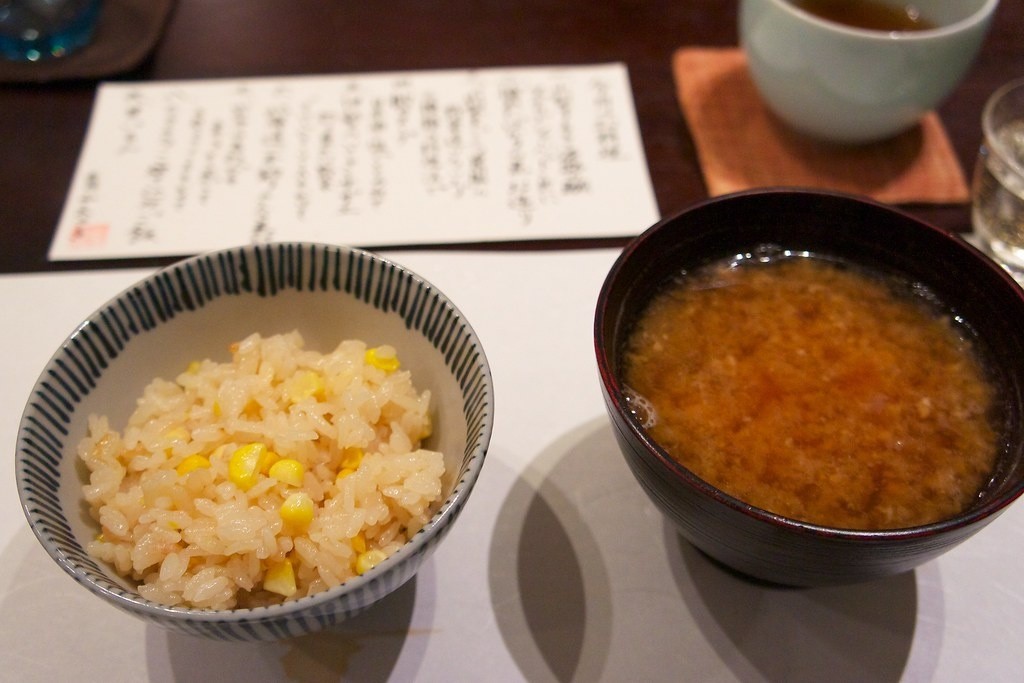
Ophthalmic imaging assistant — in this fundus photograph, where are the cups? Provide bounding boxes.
[735,0,1000,148]
[970,77,1024,271]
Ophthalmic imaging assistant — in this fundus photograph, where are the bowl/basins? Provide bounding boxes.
[15,242,496,644]
[593,186,1024,592]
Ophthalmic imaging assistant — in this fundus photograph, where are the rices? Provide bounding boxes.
[75,327,449,610]
